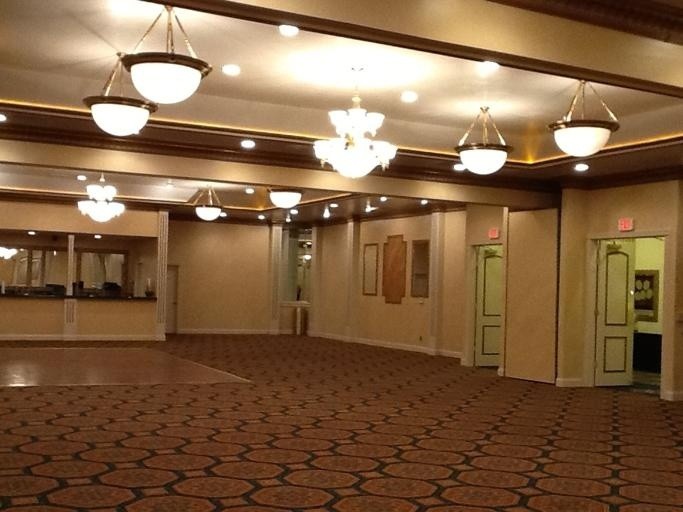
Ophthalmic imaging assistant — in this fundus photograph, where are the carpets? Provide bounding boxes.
[1,347,251,388]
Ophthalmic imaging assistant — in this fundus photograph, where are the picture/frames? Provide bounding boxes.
[634,270,659,322]
[362,243,378,297]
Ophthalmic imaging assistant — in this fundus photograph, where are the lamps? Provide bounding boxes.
[83,52,158,137]
[266,186,305,210]
[194,187,222,222]
[454,106,513,176]
[312,65,398,178]
[545,79,620,157]
[120,5,213,105]
[77,171,125,223]
